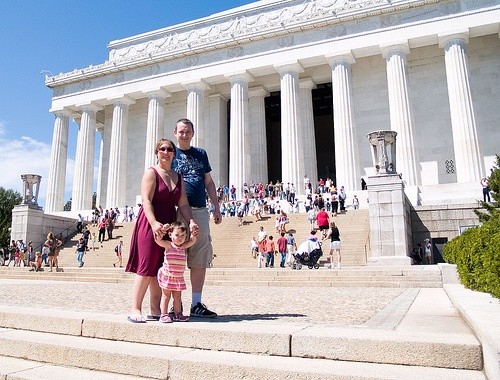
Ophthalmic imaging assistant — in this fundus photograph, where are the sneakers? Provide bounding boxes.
[159,314,172,322]
[190,302,217,317]
[173,313,189,321]
[169,302,184,315]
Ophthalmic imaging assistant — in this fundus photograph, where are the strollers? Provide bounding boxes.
[291,242,323,270]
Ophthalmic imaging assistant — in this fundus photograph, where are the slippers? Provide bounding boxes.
[147,314,160,321]
[127,316,146,323]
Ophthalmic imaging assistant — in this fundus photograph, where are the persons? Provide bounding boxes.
[113,241,123,267]
[0,238,34,267]
[28,252,42,272]
[106,219,113,239]
[90,233,97,250]
[352,195,359,210]
[410,243,423,265]
[316,209,330,240]
[156,223,197,323]
[157,119,222,317]
[91,203,141,228]
[322,221,340,269]
[98,218,105,242]
[205,175,299,228]
[480,177,491,202]
[424,238,432,265]
[308,207,316,232]
[248,210,297,268]
[74,214,84,233]
[75,238,86,267]
[125,138,199,323]
[303,174,347,218]
[82,227,91,251]
[44,231,62,272]
[41,243,58,267]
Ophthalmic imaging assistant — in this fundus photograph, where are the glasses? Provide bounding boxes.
[159,147,174,152]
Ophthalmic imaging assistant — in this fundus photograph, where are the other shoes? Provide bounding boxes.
[48,269,52,272]
[113,264,116,267]
[29,269,34,271]
